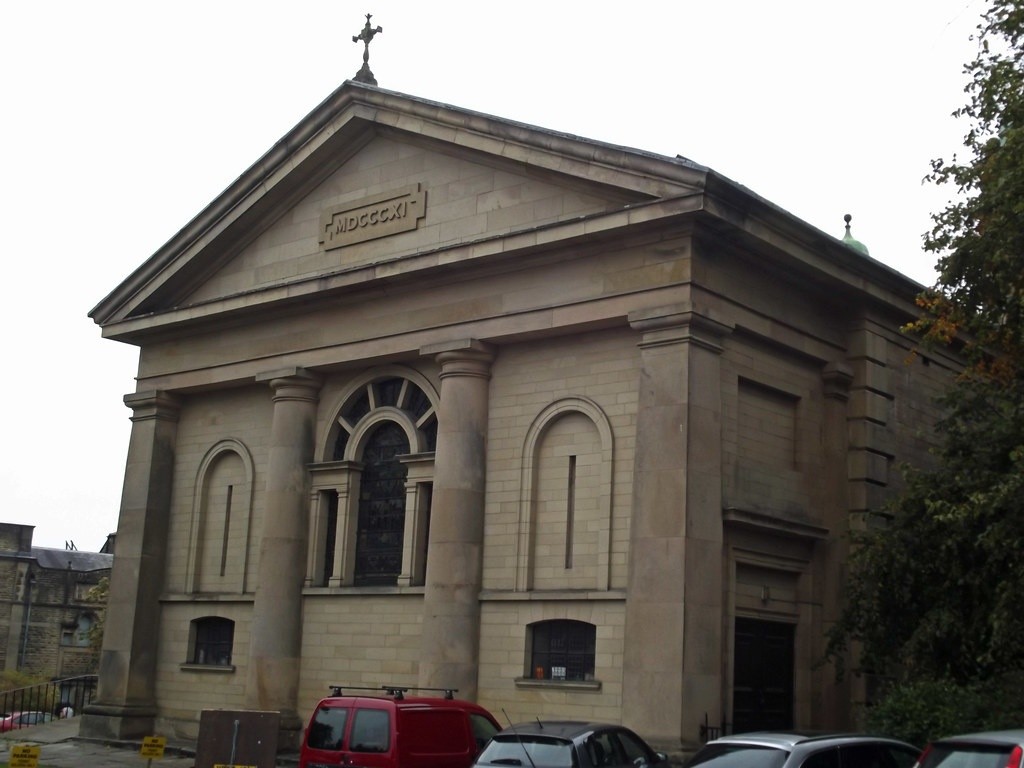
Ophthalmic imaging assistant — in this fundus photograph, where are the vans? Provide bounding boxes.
[300,685,505,768]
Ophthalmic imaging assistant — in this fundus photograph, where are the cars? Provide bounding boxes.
[914,728,1024,768]
[469,716,672,767]
[0,711,58,733]
[680,730,921,767]
